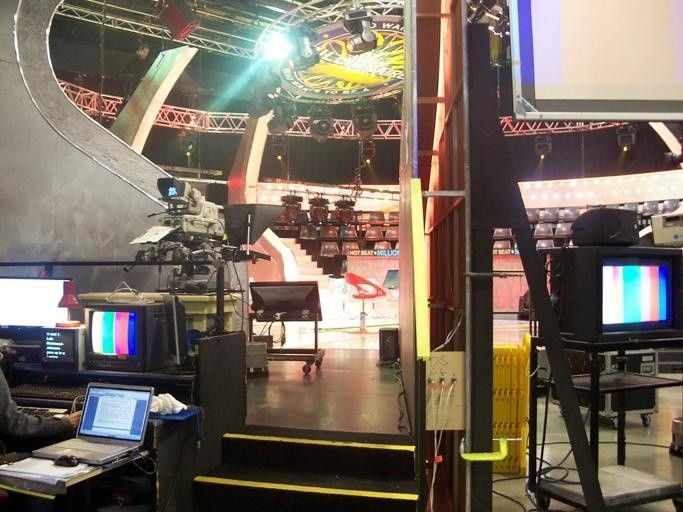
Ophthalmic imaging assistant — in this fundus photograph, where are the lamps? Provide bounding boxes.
[343,3,379,55]
[248,282,323,323]
[268,99,377,145]
[55,280,80,326]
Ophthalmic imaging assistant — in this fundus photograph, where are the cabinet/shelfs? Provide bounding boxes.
[528,335,682,510]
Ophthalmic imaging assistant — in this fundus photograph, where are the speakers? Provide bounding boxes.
[379,328,399,361]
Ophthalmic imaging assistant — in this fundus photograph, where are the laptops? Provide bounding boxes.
[32,382,155,465]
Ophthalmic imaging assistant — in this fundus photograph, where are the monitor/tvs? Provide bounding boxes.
[86,302,169,372]
[163,294,193,375]
[0,276,73,341]
[549,248,683,342]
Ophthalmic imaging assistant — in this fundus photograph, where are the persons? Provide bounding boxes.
[0,368,82,452]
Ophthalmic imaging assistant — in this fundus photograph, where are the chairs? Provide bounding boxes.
[276,211,399,256]
[344,272,385,335]
[492,200,680,250]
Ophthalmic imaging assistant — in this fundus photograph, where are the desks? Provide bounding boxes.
[76,289,240,348]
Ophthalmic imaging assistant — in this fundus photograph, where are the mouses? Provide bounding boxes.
[55,455,79,467]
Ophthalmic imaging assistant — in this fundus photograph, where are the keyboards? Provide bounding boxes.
[17,406,68,417]
